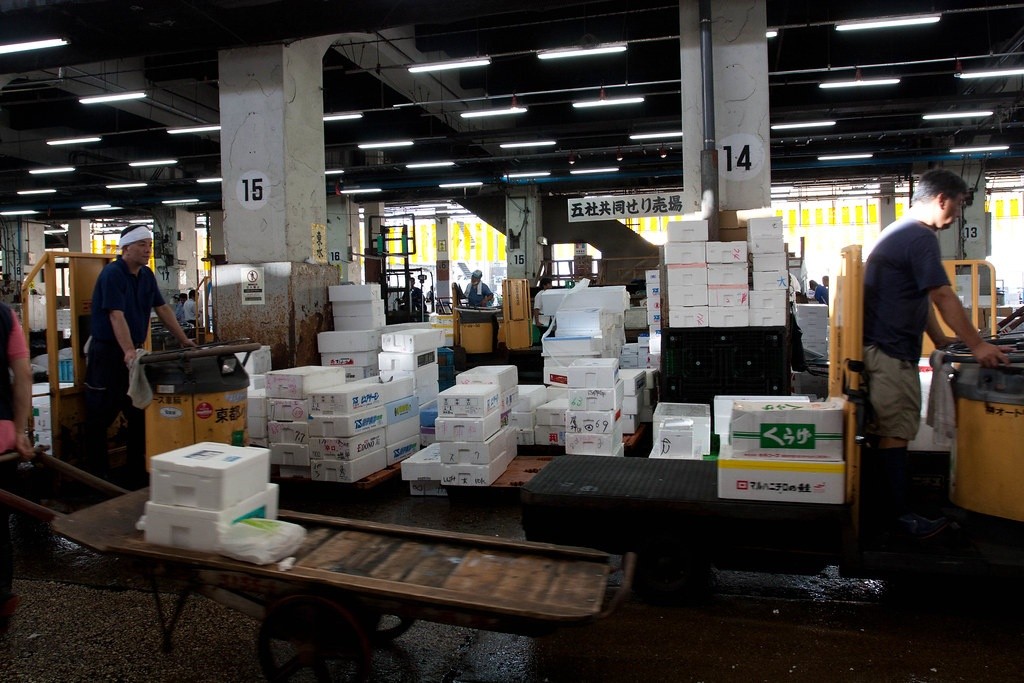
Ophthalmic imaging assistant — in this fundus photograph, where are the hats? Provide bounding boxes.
[471,269,482,277]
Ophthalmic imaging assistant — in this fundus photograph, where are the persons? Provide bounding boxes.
[809,275,829,305]
[402,278,426,314]
[457,270,493,309]
[862,169,1009,539]
[79,224,199,506]
[0,296,38,614]
[426,286,434,301]
[171,289,202,327]
[532,278,557,340]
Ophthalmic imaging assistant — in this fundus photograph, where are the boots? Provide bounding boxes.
[879,447,950,540]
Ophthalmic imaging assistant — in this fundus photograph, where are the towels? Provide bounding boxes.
[126,348,154,409]
[925,351,956,439]
[464,280,483,297]
[119,227,152,249]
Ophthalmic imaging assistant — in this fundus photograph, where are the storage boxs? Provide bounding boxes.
[28,216,865,557]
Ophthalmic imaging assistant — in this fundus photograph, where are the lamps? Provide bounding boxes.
[0,16,1022,216]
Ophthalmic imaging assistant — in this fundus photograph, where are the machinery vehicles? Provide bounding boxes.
[350,214,436,323]
